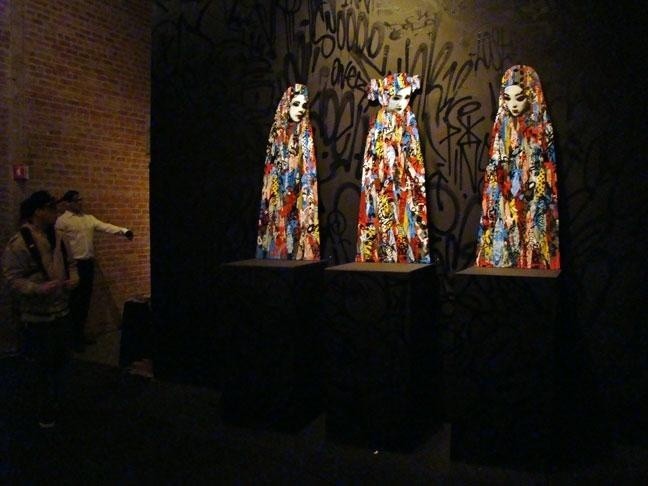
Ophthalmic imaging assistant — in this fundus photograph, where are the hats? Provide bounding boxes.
[19,190,55,218]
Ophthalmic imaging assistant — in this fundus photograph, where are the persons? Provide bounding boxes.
[255,83,322,260]
[0,192,80,429]
[57,189,134,353]
[474,64,561,269]
[353,72,431,265]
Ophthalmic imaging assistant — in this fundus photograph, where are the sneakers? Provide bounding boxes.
[73,335,97,354]
[38,412,57,428]
